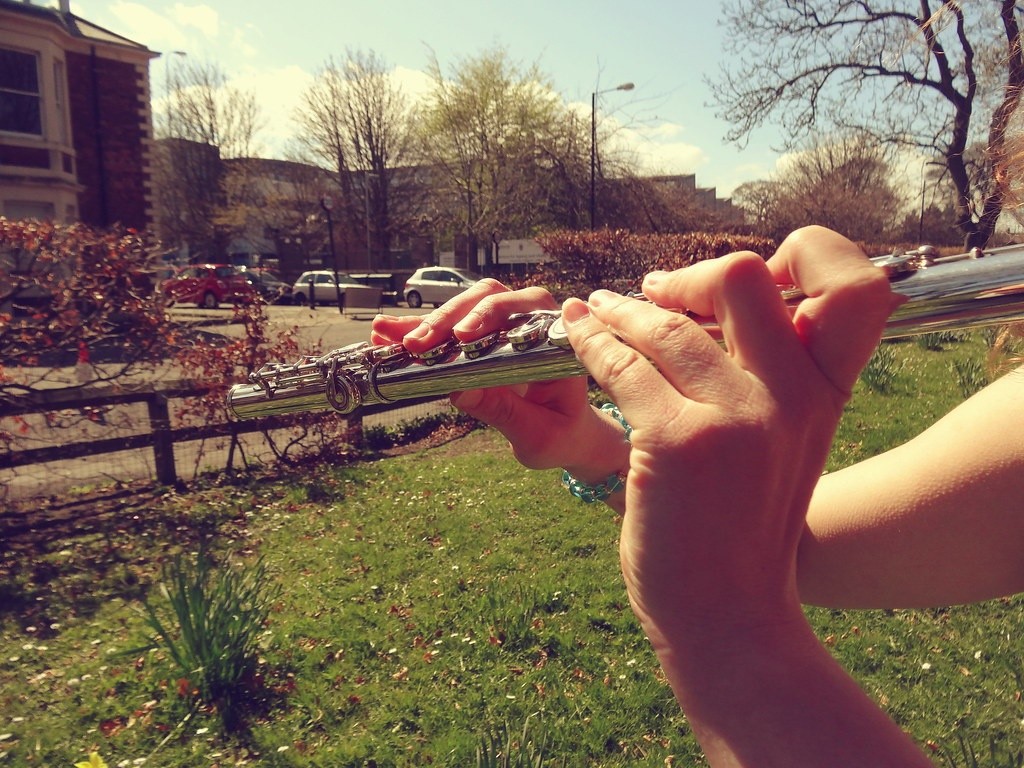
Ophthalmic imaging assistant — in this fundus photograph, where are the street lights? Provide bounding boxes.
[165,51,187,137]
[364,172,382,274]
[590,81,636,233]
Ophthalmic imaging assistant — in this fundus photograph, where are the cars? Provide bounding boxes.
[252,269,293,305]
[158,262,257,310]
[292,271,371,307]
[402,266,485,309]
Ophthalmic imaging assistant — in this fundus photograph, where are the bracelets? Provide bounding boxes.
[562,403,633,503]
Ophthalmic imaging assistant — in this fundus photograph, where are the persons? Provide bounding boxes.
[371,0,1024,767]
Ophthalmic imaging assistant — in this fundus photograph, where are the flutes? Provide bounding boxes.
[226,244,1024,420]
